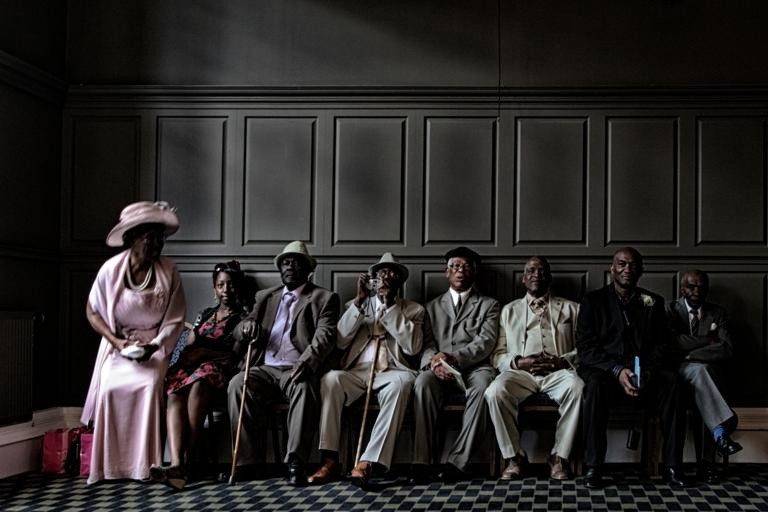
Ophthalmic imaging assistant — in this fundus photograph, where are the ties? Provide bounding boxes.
[531,299,544,317]
[689,309,701,339]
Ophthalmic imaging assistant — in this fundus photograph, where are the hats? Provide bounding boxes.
[271,239,319,274]
[444,244,483,265]
[102,198,183,250]
[366,250,411,286]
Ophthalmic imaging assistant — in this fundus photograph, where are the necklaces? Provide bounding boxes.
[120,248,156,294]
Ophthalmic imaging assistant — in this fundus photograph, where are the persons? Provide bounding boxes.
[79,201,186,484]
[149,239,742,488]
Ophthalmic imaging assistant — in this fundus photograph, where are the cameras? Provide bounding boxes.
[367,278,382,289]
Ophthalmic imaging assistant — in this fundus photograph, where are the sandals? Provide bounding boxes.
[148,459,189,492]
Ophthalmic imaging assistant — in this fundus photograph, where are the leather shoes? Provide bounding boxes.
[659,463,688,489]
[304,455,347,485]
[283,458,306,487]
[349,455,376,490]
[544,452,572,481]
[699,465,721,485]
[712,431,744,456]
[499,447,530,481]
[217,461,260,483]
[582,466,606,491]
[405,465,433,486]
[436,461,460,485]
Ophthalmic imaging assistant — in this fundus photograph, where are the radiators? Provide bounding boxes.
[0,312,46,425]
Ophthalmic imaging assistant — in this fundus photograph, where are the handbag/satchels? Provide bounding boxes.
[39,425,71,477]
[77,427,93,477]
